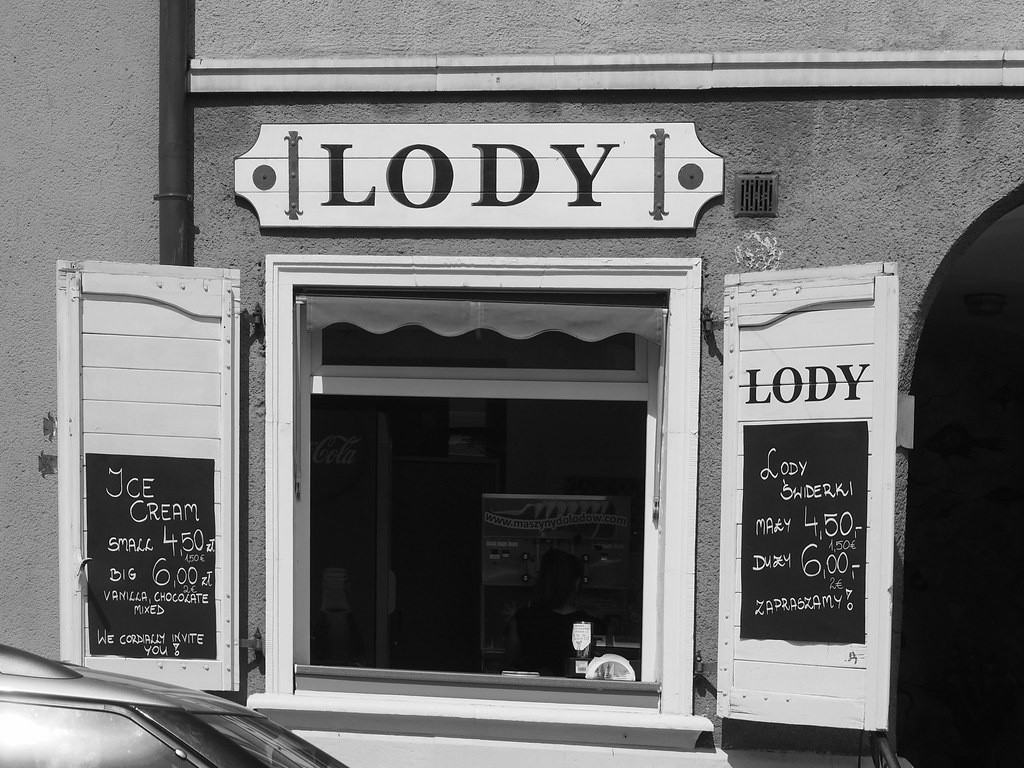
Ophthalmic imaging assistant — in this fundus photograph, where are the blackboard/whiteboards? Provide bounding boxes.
[738,420,869,641]
[86,451,218,661]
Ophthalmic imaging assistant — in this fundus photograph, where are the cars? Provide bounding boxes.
[0,643,351,768]
